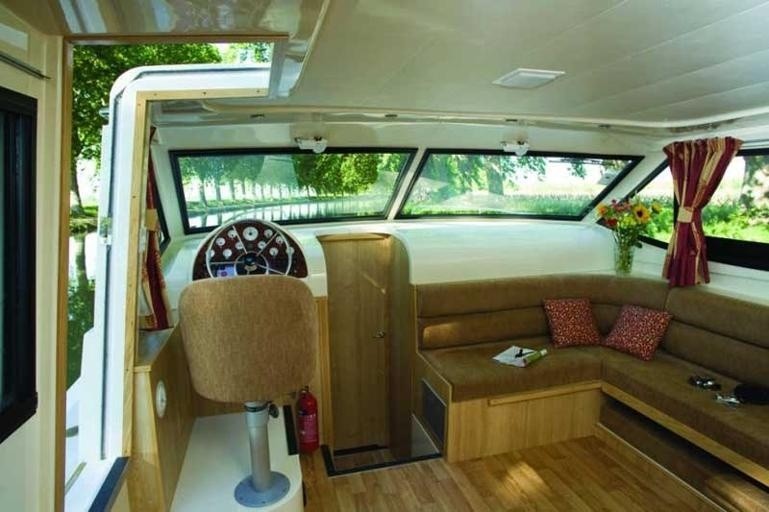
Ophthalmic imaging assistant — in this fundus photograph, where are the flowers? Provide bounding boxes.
[592,190,662,269]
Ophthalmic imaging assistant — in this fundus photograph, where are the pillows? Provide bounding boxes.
[541,297,603,348]
[602,303,674,362]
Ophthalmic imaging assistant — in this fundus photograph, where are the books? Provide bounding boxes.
[492,345,548,370]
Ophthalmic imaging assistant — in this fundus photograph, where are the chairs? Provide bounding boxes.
[174,277,320,510]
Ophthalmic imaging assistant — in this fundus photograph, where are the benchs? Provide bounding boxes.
[594,287,769,498]
[409,268,669,461]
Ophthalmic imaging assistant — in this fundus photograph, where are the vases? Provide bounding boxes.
[612,245,634,277]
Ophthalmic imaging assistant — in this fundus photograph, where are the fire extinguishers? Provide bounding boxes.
[296,385,320,457]
